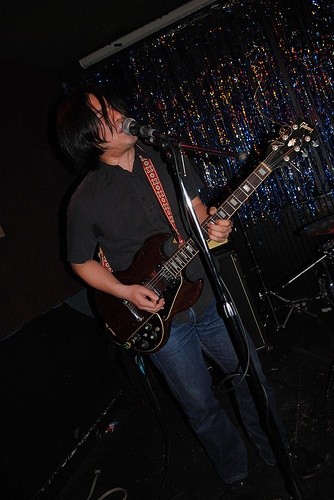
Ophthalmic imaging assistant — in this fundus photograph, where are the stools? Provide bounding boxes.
[300,213,334,311]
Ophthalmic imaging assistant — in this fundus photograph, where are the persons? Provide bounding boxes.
[58,84,324,500]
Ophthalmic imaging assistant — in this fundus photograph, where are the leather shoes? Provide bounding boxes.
[288,445,328,480]
[226,478,257,500]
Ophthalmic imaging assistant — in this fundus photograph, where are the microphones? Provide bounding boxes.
[123,118,178,143]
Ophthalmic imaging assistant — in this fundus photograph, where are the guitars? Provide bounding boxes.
[87,115,321,356]
